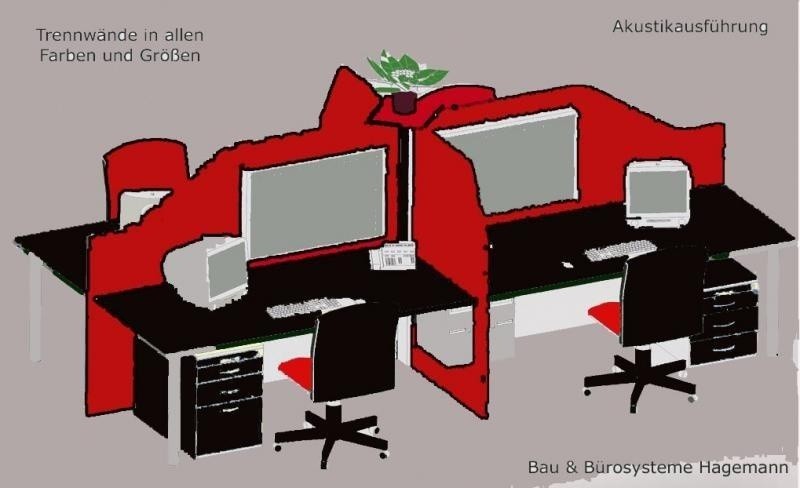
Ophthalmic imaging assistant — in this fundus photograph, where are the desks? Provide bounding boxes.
[13,222,117,363]
[488,183,796,369]
[92,243,477,460]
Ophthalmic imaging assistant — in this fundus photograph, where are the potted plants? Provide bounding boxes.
[366,47,450,116]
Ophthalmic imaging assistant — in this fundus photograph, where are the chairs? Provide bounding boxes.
[102,138,188,225]
[274,302,400,470]
[583,244,706,416]
